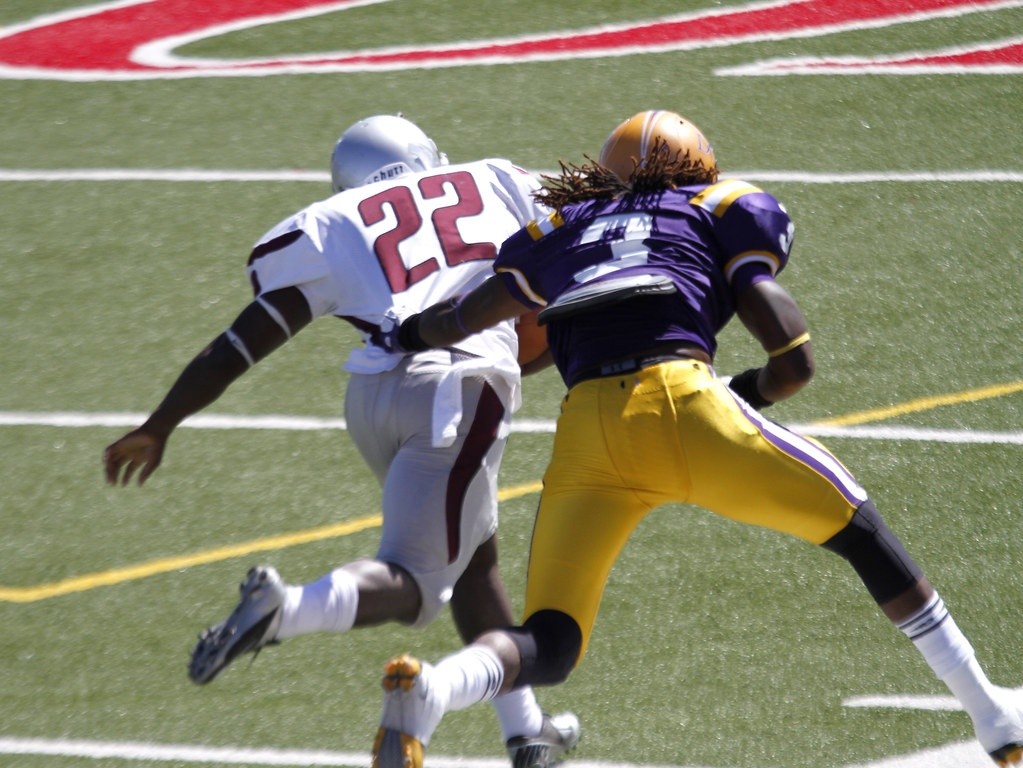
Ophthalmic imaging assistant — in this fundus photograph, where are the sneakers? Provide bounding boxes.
[972,684,1023,767]
[187,566,286,685]
[505,711,580,768]
[372,655,424,768]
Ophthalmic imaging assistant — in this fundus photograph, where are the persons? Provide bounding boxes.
[102,114,581,768]
[355,110,1023,768]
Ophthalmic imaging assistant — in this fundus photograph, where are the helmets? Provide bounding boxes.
[599,108,716,180]
[331,116,448,198]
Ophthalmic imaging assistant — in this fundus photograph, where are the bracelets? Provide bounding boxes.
[398,313,431,352]
[728,369,775,411]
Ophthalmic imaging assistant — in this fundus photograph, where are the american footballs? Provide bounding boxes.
[515,310,553,377]
